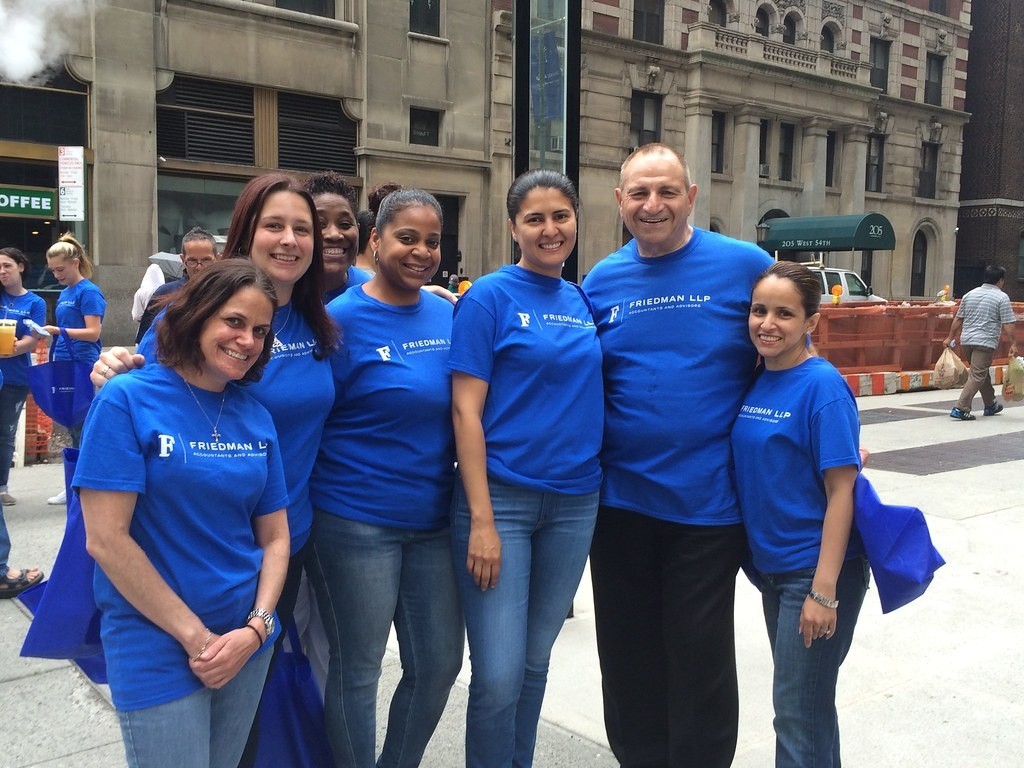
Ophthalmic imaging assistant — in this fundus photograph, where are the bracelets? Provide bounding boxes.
[192,627,211,662]
[809,589,839,609]
[245,624,263,647]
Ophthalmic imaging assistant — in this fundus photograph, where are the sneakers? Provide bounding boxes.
[984,403,1003,416]
[950,407,976,420]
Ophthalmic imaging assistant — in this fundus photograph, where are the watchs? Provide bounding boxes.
[247,609,275,637]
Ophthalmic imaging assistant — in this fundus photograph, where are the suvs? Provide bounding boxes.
[807,264,888,304]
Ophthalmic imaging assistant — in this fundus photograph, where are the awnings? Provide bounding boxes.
[758,212,896,301]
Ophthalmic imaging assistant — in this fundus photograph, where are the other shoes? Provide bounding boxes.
[1,494,17,506]
[47,490,66,504]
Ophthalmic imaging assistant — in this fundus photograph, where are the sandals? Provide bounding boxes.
[0,568,44,599]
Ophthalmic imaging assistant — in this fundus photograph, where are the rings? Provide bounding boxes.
[821,628,831,634]
[102,366,109,377]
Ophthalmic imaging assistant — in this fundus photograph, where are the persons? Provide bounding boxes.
[446,169,603,768]
[31,230,108,505]
[308,189,470,768]
[579,142,869,768]
[730,262,872,768]
[71,259,294,768]
[132,226,221,346]
[942,265,1018,420]
[0,246,46,507]
[0,497,46,599]
[89,172,340,768]
[301,170,462,308]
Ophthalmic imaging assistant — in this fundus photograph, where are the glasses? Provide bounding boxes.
[185,256,216,268]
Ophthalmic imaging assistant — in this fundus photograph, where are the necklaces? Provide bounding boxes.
[5,290,21,308]
[182,378,226,444]
[272,299,294,347]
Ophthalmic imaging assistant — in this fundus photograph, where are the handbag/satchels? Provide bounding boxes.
[26,327,96,428]
[244,611,337,768]
[1002,354,1024,401]
[933,346,968,388]
